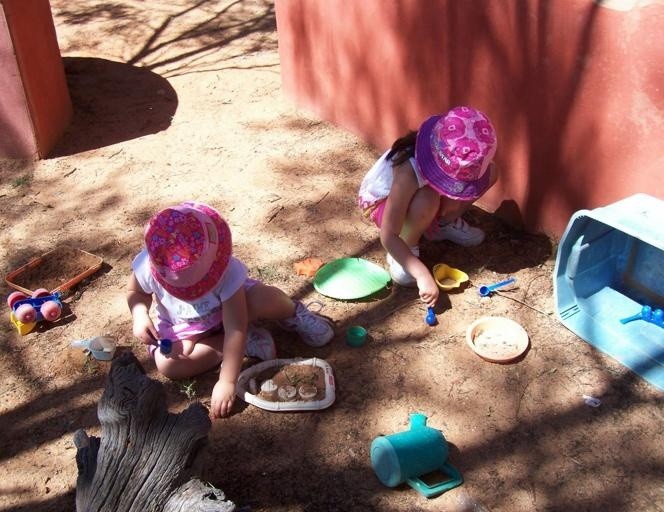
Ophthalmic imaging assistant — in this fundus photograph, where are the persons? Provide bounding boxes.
[124,200,337,422]
[356,104,500,311]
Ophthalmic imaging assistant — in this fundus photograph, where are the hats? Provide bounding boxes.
[416,105,499,202]
[146,200,233,301]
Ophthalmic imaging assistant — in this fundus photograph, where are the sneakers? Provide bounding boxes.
[423,217,486,246]
[243,322,276,361]
[277,300,334,347]
[386,246,421,288]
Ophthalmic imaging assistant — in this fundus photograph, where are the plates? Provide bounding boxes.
[313,257,391,301]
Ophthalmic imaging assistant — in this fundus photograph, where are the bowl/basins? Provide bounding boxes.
[467,317,529,362]
